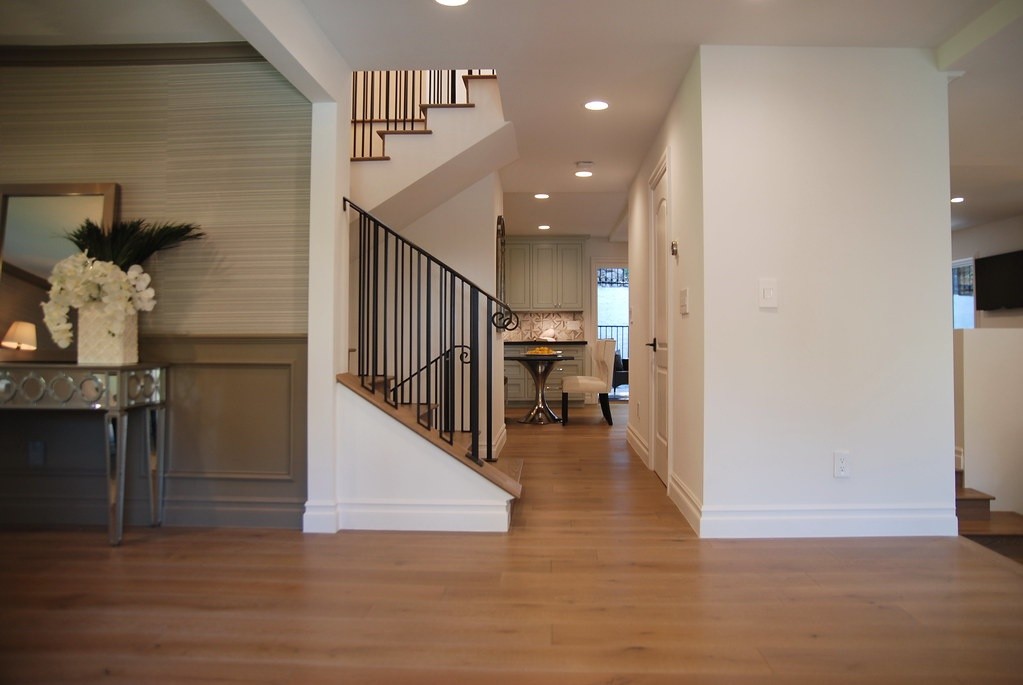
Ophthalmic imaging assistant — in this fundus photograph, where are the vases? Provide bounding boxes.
[78,301,139,366]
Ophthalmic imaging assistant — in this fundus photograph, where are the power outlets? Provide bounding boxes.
[834,451,852,478]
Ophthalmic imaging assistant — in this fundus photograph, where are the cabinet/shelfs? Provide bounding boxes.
[502,233,592,311]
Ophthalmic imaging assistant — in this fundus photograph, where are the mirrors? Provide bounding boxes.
[951,256,976,329]
[0,182,121,364]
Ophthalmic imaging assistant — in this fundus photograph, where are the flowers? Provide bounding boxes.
[39,247,156,348]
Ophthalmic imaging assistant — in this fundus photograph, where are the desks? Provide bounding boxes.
[504,355,574,424]
[0,362,170,545]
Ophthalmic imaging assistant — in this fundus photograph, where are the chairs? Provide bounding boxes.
[560,338,616,425]
[613,354,629,391]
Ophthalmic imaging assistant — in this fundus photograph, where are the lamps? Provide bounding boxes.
[2,321,37,350]
